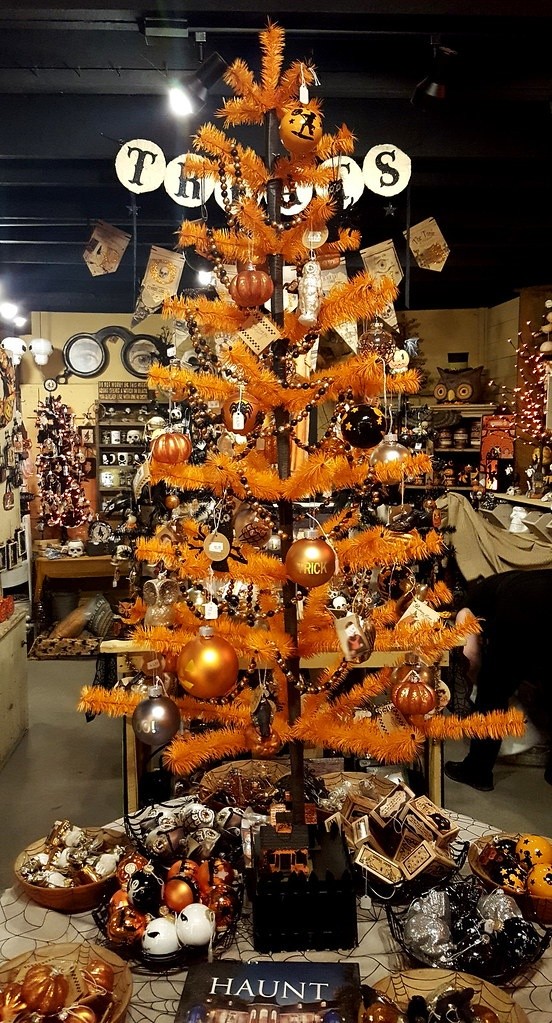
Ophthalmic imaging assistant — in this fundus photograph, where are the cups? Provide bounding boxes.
[101,472,113,488]
[134,452,147,465]
[111,431,121,445]
[102,452,116,465]
[118,452,134,466]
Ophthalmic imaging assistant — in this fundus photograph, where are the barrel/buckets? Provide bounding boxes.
[50,592,74,623]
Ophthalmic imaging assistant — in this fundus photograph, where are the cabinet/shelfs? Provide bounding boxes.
[93,399,497,538]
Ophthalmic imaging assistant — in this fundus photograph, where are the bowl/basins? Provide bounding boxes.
[358,969,531,1023]
[0,942,134,1023]
[14,828,133,912]
[201,760,291,802]
[317,771,398,816]
[468,832,552,924]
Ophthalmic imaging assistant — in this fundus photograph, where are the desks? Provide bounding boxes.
[99,634,449,816]
[0,795,552,1023]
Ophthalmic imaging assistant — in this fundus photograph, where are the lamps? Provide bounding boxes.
[172,43,229,117]
[410,36,457,113]
